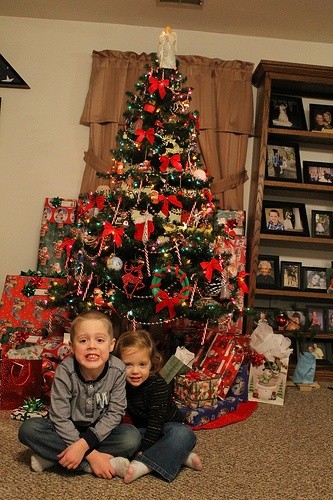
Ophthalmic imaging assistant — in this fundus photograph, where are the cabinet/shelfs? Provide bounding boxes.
[238,58,333,381]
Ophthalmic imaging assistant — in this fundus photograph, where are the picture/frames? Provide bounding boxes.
[255,254,280,289]
[311,210,333,239]
[305,304,326,332]
[302,160,333,187]
[261,200,310,237]
[281,261,302,290]
[309,103,333,134]
[254,307,280,329]
[326,306,333,333]
[301,266,328,293]
[268,95,307,130]
[284,309,307,331]
[298,339,328,366]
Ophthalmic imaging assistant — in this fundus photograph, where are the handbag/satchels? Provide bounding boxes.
[292,351,316,385]
[0,358,46,411]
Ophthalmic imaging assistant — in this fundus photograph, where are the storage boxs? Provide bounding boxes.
[0,197,289,429]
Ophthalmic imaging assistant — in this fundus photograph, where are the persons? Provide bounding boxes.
[256,102,333,359]
[113,330,202,484]
[18,312,142,479]
